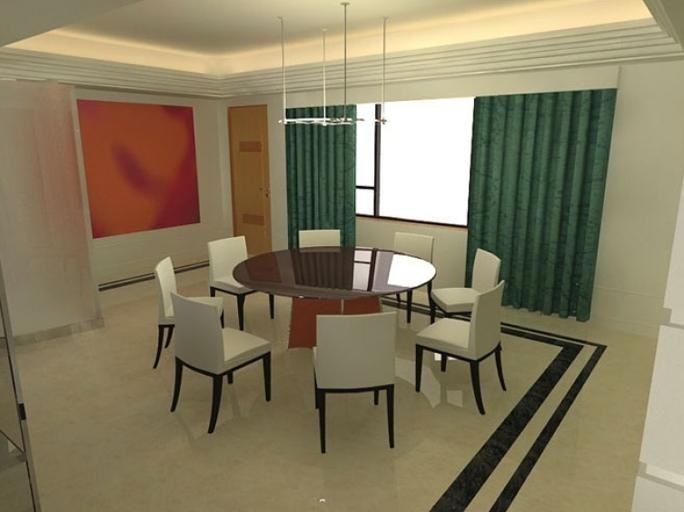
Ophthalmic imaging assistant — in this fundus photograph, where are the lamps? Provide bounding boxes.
[276,0,390,126]
